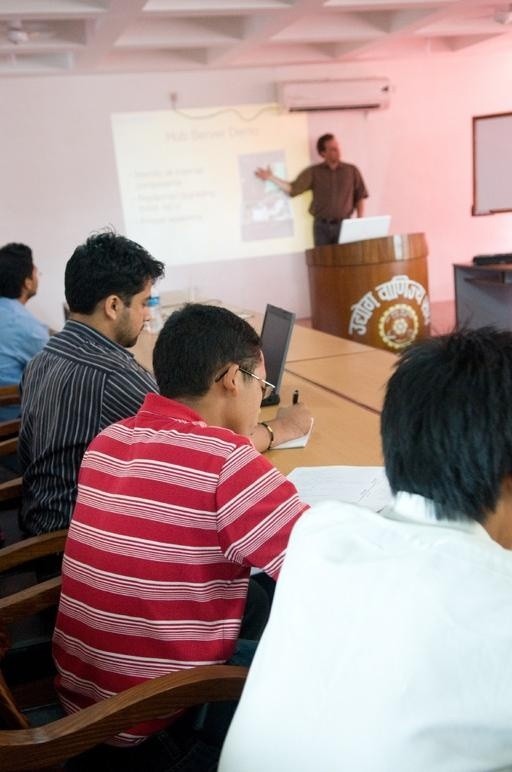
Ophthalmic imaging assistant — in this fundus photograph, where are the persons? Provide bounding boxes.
[218,326,512,772]
[17,232,311,619]
[51,303,311,772]
[0,243,59,475]
[255,133,368,246]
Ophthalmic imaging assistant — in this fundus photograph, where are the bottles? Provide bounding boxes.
[146,284,163,334]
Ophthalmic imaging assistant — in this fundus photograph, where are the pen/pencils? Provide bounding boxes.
[293,390,298,405]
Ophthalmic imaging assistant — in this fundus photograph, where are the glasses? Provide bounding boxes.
[215,366,277,402]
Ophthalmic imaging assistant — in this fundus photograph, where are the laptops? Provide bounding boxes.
[337,215,392,245]
[260,303,296,407]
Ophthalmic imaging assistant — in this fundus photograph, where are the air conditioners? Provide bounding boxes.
[274,77,395,113]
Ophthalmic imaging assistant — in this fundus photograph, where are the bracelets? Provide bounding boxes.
[257,421,274,450]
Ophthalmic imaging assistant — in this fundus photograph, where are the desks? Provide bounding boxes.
[125,298,412,513]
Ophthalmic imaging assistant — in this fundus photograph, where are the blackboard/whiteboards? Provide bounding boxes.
[472,112,512,216]
